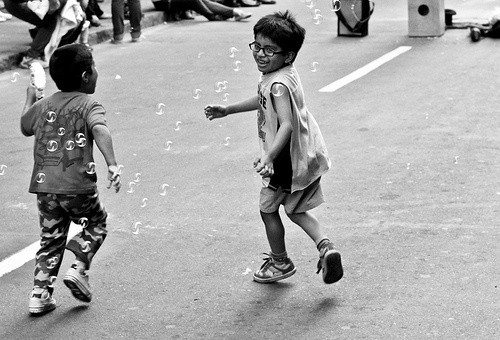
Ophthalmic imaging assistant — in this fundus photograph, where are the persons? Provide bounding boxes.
[151,0,276,22]
[203,9,344,284]
[21,43,125,314]
[4,0,104,68]
[110,0,146,44]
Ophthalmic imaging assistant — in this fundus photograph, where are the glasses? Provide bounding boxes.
[249,41,284,57]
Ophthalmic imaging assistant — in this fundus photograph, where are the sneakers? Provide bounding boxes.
[234,12,252,22]
[131,36,140,43]
[316,244,343,284]
[252,252,297,284]
[20,56,50,69]
[28,287,57,314]
[63,264,93,302]
[109,37,123,44]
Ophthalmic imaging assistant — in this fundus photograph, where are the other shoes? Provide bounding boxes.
[240,0,276,7]
